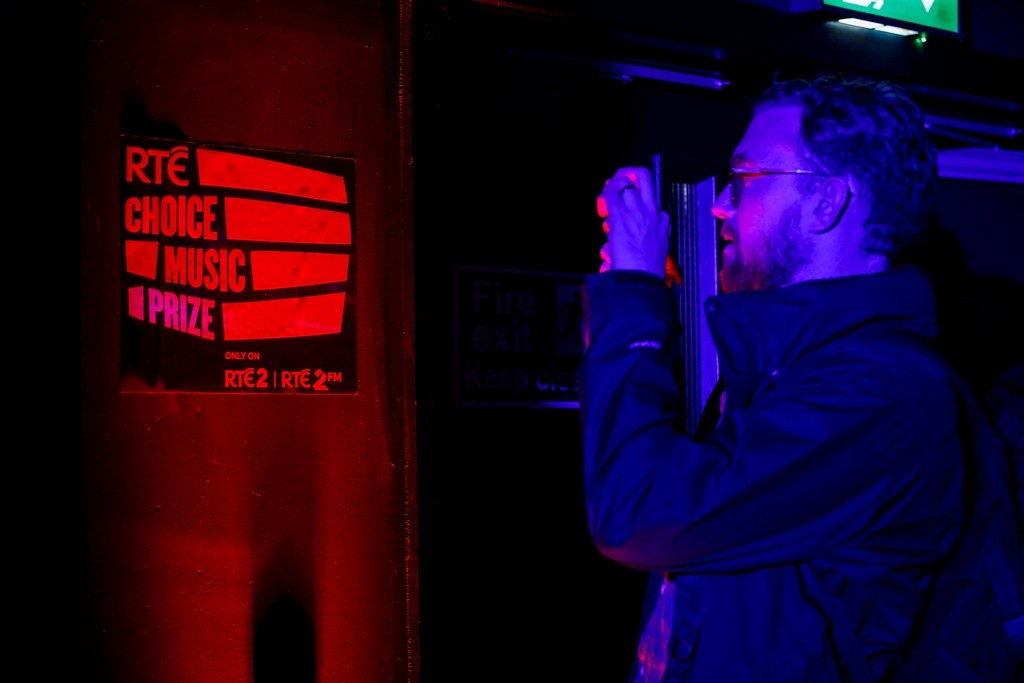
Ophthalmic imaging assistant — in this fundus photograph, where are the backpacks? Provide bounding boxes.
[862,330,1024,683]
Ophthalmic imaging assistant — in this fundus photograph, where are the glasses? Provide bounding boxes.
[727,169,841,208]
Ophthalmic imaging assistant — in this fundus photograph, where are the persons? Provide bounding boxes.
[582,75,964,683]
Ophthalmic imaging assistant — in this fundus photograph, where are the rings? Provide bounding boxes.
[619,184,635,196]
